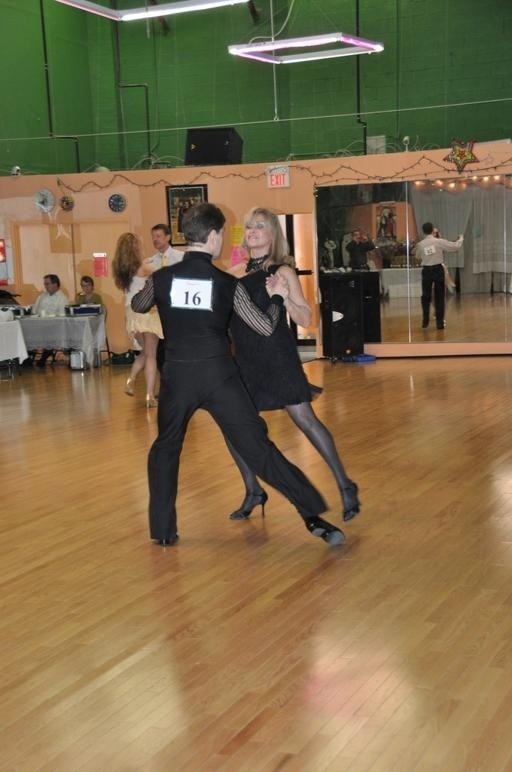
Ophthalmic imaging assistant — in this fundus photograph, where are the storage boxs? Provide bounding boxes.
[69,350,86,369]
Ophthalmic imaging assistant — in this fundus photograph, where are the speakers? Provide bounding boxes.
[352,272,380,342]
[185,127,243,164]
[319,272,364,364]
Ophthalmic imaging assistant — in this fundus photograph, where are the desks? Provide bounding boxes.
[14,315,105,366]
[0,319,28,383]
[378,268,426,300]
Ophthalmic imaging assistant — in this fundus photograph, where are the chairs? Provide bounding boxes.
[101,303,111,359]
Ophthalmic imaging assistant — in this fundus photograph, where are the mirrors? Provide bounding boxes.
[312,173,511,346]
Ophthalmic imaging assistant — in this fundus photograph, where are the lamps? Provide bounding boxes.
[56,0,251,20]
[11,165,21,176]
[225,1,387,64]
[403,135,410,151]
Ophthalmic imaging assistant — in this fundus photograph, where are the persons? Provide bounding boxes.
[111,231,165,407]
[145,223,186,271]
[409,227,459,328]
[22,274,68,368]
[0,289,22,309]
[131,202,348,549]
[324,237,336,266]
[346,230,375,271]
[74,275,101,306]
[216,205,365,521]
[414,222,465,329]
[376,207,396,238]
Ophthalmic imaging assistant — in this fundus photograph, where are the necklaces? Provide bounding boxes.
[245,255,269,274]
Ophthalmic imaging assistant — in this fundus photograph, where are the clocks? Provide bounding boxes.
[107,192,126,212]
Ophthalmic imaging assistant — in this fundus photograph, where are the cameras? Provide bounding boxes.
[357,235,367,240]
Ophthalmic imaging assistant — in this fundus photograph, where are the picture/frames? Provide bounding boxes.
[165,184,207,246]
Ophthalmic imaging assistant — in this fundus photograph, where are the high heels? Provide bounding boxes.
[145,393,158,408]
[230,489,268,520]
[340,483,360,521]
[124,378,135,397]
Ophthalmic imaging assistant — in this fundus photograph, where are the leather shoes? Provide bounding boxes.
[306,517,344,546]
[160,534,178,545]
